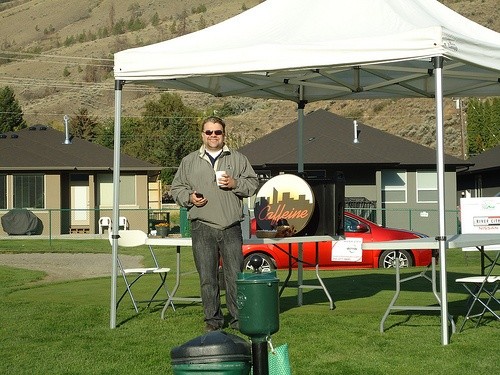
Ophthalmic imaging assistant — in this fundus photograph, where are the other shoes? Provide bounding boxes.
[231,321,239,330]
[205,324,217,332]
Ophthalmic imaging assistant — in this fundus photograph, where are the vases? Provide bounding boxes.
[156,227,168,237]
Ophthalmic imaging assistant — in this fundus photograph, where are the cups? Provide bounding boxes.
[215,171,225,186]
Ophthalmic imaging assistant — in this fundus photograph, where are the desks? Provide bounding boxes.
[146,235,346,320]
[362,234,500,333]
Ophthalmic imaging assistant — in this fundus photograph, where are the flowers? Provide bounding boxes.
[155,223,169,228]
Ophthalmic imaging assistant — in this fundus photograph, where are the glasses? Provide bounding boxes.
[203,130,224,135]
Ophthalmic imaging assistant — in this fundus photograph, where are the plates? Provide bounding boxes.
[255,231,278,238]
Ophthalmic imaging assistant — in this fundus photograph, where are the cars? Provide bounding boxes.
[219,211,439,272]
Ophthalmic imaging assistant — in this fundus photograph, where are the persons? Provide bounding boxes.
[171,116,259,332]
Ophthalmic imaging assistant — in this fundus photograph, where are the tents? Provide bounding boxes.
[110,0,500,331]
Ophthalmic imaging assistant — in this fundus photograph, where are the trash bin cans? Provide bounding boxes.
[154,225,169,237]
[169,331,252,374]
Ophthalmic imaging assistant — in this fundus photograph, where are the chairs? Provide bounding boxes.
[98,217,111,234]
[109,230,176,317]
[119,216,127,231]
[455,251,500,333]
